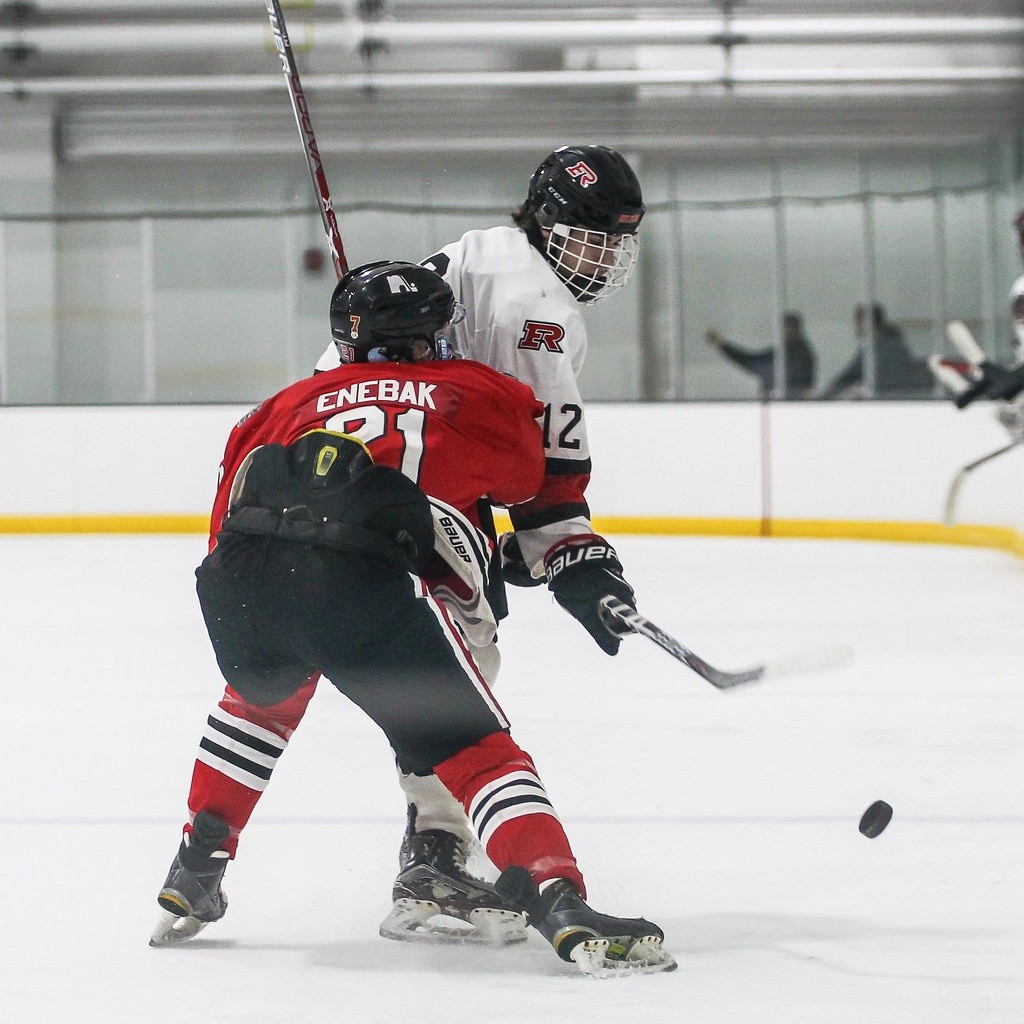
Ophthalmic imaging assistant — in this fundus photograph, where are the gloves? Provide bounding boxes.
[542,533,638,656]
[953,360,1024,408]
[498,532,548,588]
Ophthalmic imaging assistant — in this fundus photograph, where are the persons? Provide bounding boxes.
[148,260,679,980]
[820,302,914,400]
[705,309,815,397]
[928,209,1024,445]
[312,143,648,946]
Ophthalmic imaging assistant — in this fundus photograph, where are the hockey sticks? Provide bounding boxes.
[263,1,350,281]
[597,594,766,689]
[941,440,1024,539]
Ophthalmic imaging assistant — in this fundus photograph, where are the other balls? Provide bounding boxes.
[859,799,894,840]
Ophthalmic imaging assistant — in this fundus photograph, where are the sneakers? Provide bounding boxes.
[148,810,228,948]
[379,804,530,948]
[494,864,679,979]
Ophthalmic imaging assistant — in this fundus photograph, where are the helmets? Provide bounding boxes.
[329,253,469,366]
[527,143,645,307]
